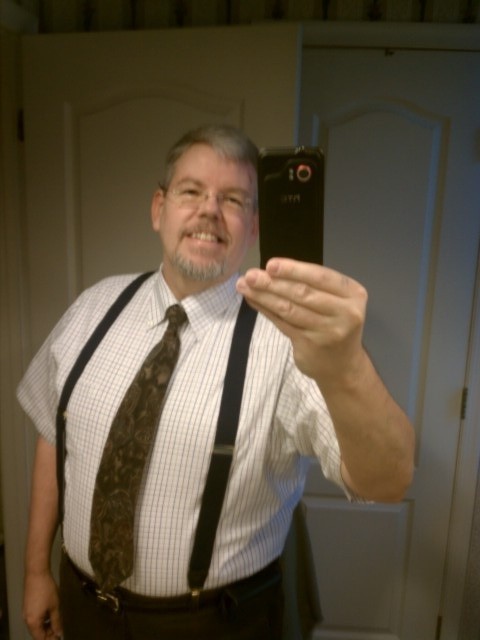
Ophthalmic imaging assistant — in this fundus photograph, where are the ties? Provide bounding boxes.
[88,307,186,594]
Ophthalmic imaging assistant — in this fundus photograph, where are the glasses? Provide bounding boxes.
[162,183,255,209]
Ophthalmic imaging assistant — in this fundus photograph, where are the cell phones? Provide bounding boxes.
[256,142,325,274]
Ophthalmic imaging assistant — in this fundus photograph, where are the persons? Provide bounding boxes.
[16,126,416,640]
